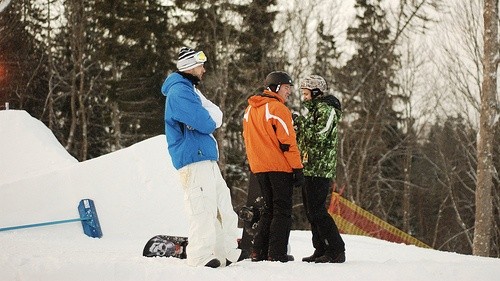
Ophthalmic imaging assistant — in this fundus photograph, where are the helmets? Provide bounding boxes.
[300,75,327,97]
[265,71,295,90]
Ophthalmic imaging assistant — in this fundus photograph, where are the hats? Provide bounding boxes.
[176,47,204,72]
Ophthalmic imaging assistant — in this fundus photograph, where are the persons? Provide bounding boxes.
[161,46,249,268]
[290,72,347,263]
[243,69,307,263]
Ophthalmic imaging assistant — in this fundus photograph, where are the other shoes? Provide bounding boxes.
[206,258,221,268]
[301,253,317,262]
[315,251,346,263]
[250,248,268,261]
[268,254,294,262]
[226,249,248,266]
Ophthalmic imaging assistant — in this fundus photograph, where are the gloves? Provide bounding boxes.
[292,168,306,188]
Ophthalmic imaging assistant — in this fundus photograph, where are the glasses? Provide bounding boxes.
[194,51,207,63]
